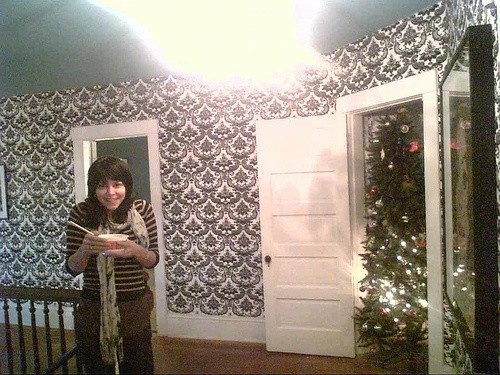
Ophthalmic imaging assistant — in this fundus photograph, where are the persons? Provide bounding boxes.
[62,154,160,375]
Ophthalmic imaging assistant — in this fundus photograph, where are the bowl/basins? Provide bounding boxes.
[98,234,129,256]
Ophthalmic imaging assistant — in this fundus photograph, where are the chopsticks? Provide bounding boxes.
[67,221,96,236]
